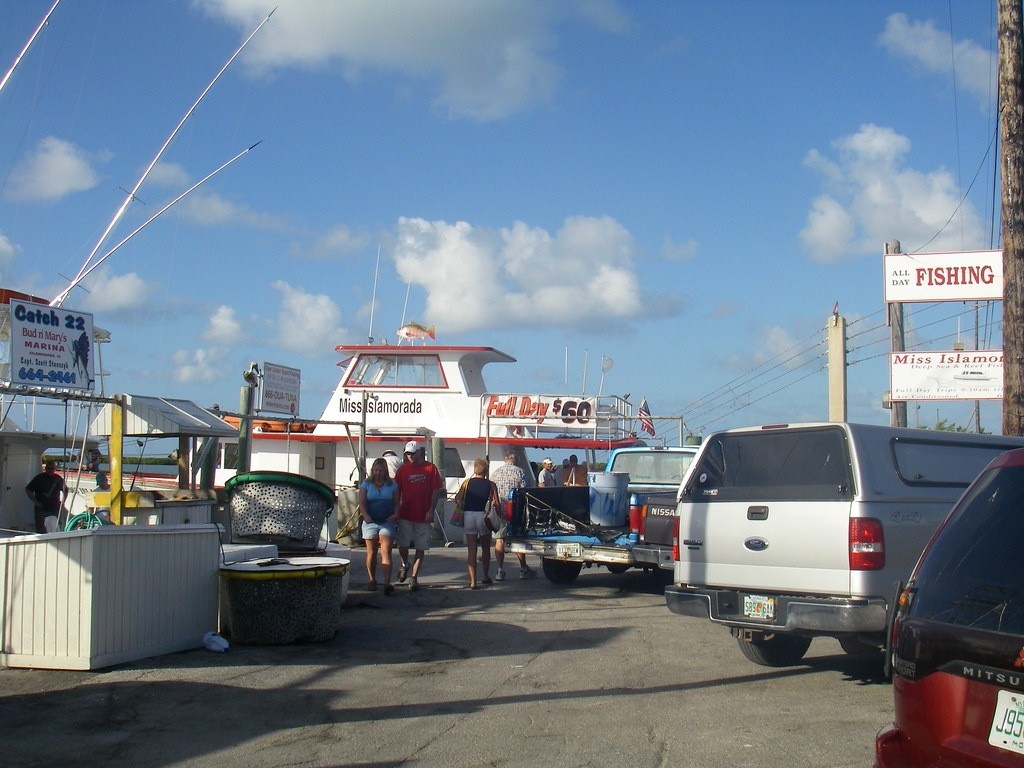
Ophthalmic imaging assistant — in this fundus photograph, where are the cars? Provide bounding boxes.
[872,449,1024,768]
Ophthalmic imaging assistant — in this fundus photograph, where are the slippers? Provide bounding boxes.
[482,578,493,583]
[468,582,476,589]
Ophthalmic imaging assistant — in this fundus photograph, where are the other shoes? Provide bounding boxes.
[384,584,393,596]
[368,580,378,591]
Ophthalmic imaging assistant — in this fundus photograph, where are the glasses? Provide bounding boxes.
[47,467,55,470]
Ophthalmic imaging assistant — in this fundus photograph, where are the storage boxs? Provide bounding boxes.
[643,497,678,546]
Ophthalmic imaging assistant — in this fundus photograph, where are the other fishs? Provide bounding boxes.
[396,322,435,340]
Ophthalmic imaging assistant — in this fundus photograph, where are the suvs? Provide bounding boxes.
[662,418,1024,664]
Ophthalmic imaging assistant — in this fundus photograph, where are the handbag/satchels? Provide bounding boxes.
[450,478,470,528]
[484,481,502,534]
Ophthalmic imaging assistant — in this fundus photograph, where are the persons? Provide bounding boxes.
[455,458,501,589]
[381,449,403,479]
[360,458,400,595]
[537,457,555,487]
[554,458,569,487]
[392,440,444,588]
[77,441,103,473]
[563,454,587,487]
[489,447,537,581]
[25,459,68,533]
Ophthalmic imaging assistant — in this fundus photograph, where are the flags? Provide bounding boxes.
[638,400,655,437]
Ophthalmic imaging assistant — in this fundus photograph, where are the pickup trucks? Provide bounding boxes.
[503,443,704,584]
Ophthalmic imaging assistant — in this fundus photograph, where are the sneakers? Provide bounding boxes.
[398,560,410,583]
[409,576,419,593]
[496,570,506,581]
[519,565,537,579]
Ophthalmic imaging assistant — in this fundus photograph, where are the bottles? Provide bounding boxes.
[203,632,229,654]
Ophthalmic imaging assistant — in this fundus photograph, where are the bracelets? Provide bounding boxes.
[362,512,366,516]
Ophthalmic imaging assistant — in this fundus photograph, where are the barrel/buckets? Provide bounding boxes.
[587,471,631,527]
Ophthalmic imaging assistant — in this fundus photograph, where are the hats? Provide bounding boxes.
[543,457,553,463]
[404,441,421,453]
[563,459,570,465]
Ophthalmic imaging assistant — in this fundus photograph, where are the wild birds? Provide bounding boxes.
[243,361,259,387]
